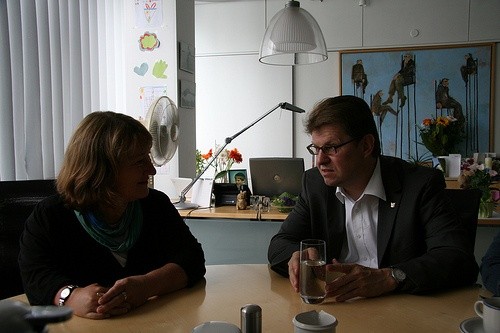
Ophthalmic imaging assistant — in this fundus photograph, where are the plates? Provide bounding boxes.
[459,316,483,333]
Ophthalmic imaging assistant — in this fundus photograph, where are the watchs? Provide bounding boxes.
[391,265,406,290]
[57,284,79,306]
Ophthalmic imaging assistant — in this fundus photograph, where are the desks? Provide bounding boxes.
[1,264,494,333]
[178,206,500,227]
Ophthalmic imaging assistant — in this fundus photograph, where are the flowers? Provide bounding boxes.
[202,140,243,182]
[415,114,471,155]
[461,158,498,218]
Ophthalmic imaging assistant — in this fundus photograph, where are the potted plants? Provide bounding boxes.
[196,149,206,177]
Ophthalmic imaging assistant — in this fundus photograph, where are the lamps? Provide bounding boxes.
[259,0,328,66]
[359,0,368,7]
[174,102,305,209]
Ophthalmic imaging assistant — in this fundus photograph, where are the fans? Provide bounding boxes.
[145,96,180,189]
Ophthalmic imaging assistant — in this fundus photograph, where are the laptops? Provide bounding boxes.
[250,158,305,196]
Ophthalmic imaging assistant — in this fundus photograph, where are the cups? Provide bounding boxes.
[298,240,328,305]
[474,298,500,333]
[292,310,338,333]
[484,153,496,168]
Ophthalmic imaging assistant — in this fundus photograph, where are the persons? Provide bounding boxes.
[478,232,500,295]
[19,112,207,319]
[234,173,246,189]
[267,96,480,302]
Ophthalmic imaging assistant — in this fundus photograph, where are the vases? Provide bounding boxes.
[210,170,229,207]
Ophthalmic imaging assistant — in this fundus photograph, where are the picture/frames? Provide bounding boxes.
[338,42,496,181]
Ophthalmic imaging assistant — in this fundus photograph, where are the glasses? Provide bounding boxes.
[306,131,368,155]
